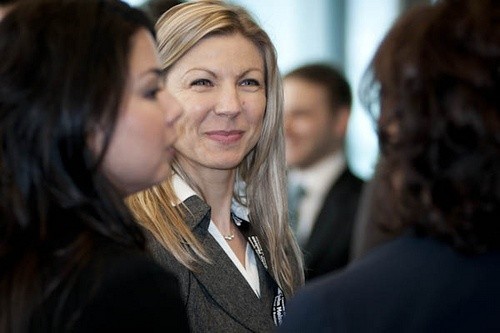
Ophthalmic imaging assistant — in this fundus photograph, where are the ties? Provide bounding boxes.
[286,184,306,232]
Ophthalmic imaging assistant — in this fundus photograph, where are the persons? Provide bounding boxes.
[281,64,369,283]
[0,0,192,333]
[123,0,305,333]
[278,0,500,333]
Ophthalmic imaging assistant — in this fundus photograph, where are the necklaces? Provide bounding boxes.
[223,228,236,241]
